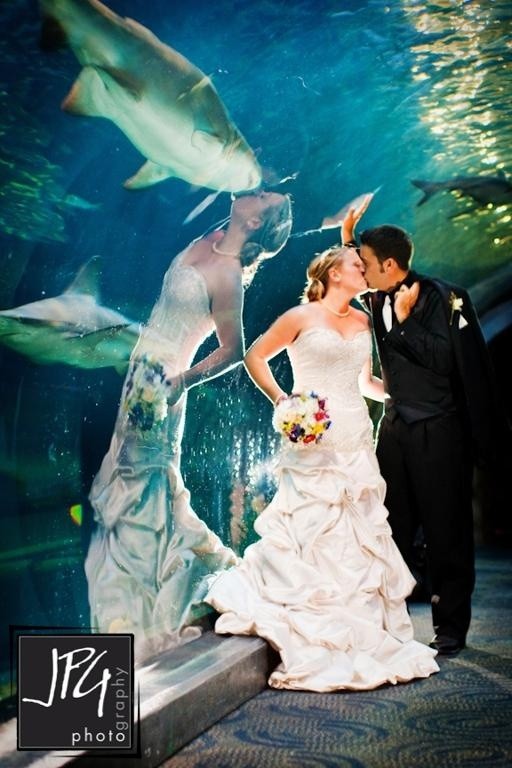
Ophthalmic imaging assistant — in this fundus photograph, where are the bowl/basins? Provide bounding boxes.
[319,296,350,317]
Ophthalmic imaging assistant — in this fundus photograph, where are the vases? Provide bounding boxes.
[272,390,331,446]
[124,352,172,440]
[448,291,463,326]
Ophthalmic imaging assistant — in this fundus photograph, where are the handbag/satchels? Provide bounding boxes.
[274,393,287,408]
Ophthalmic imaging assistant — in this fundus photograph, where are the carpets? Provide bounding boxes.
[381,295,395,333]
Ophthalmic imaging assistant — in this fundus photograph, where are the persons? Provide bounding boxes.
[340,193,493,654]
[86,191,293,672]
[205,245,442,695]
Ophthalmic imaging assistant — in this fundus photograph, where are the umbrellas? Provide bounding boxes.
[430,633,466,658]
